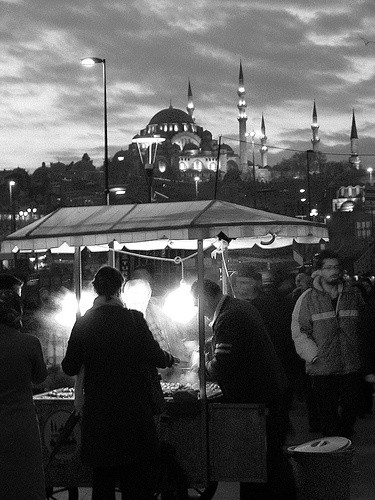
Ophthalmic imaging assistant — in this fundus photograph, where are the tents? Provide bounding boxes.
[0,201,330,486]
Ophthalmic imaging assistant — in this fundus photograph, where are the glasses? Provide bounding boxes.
[321,265,344,271]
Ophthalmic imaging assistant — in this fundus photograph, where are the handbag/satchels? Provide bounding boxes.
[147,367,167,415]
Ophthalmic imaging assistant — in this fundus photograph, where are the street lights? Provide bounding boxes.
[131,134,166,202]
[244,131,256,182]
[9,179,16,233]
[80,56,110,205]
[194,176,200,199]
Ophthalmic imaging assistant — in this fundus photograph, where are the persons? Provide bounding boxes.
[290,249,366,440]
[3,274,24,334]
[61,265,181,500]
[0,290,49,500]
[189,278,295,500]
[220,262,375,433]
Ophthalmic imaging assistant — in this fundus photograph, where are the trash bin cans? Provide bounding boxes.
[288,436,355,500]
